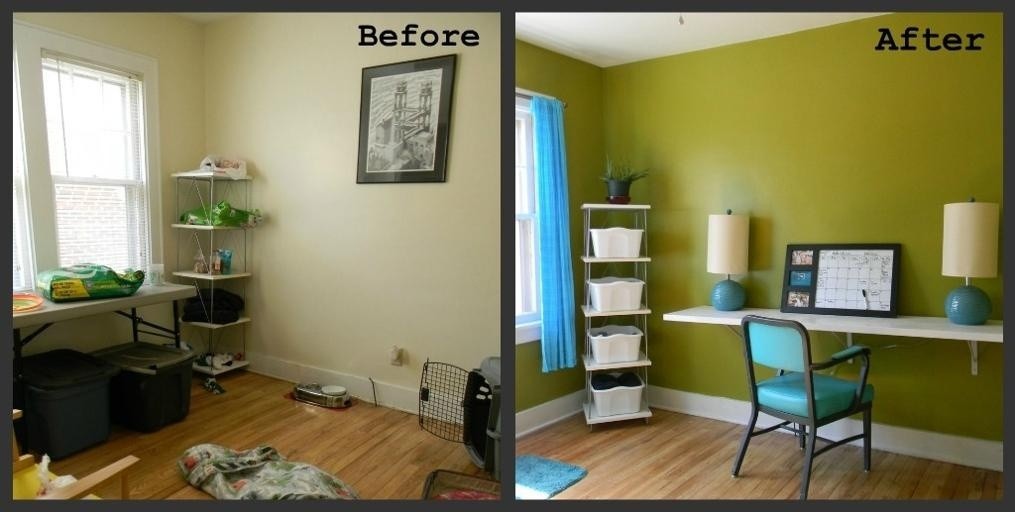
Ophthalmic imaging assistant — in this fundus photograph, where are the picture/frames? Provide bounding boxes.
[357,55,458,183]
[781,243,900,317]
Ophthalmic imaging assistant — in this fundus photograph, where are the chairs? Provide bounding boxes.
[13,409,141,500]
[734,316,875,499]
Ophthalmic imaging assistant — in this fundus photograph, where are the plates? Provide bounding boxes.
[12,293,44,312]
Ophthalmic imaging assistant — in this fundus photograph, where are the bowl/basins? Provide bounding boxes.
[322,385,346,396]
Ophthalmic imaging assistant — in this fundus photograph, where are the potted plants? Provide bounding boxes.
[601,158,645,202]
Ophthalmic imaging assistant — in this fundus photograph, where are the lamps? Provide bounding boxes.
[942,198,999,325]
[706,209,751,310]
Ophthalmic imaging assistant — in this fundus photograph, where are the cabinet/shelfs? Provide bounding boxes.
[173,172,252,391]
[581,204,653,432]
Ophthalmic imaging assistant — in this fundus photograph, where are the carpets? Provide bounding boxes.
[516,451,586,500]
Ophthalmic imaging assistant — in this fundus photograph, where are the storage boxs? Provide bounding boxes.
[590,227,645,258]
[20,348,121,460]
[587,276,645,312]
[592,372,645,417]
[89,341,197,433]
[587,325,644,363]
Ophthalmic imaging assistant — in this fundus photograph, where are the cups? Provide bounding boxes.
[147,264,165,287]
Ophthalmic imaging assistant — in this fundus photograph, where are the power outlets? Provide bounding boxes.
[391,348,403,366]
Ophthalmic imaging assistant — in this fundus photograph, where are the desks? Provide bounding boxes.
[13,281,196,454]
